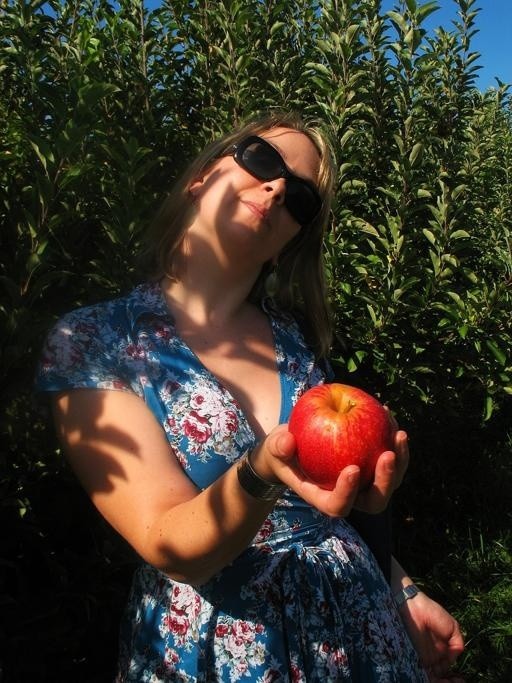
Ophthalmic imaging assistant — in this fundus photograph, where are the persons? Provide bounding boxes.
[30,114,467,683]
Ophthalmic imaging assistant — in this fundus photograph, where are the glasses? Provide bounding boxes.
[216,134,323,229]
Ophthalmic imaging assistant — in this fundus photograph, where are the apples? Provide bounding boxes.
[288,383,395,491]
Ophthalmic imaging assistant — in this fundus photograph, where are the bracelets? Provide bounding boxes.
[391,585,419,609]
[236,447,289,502]
